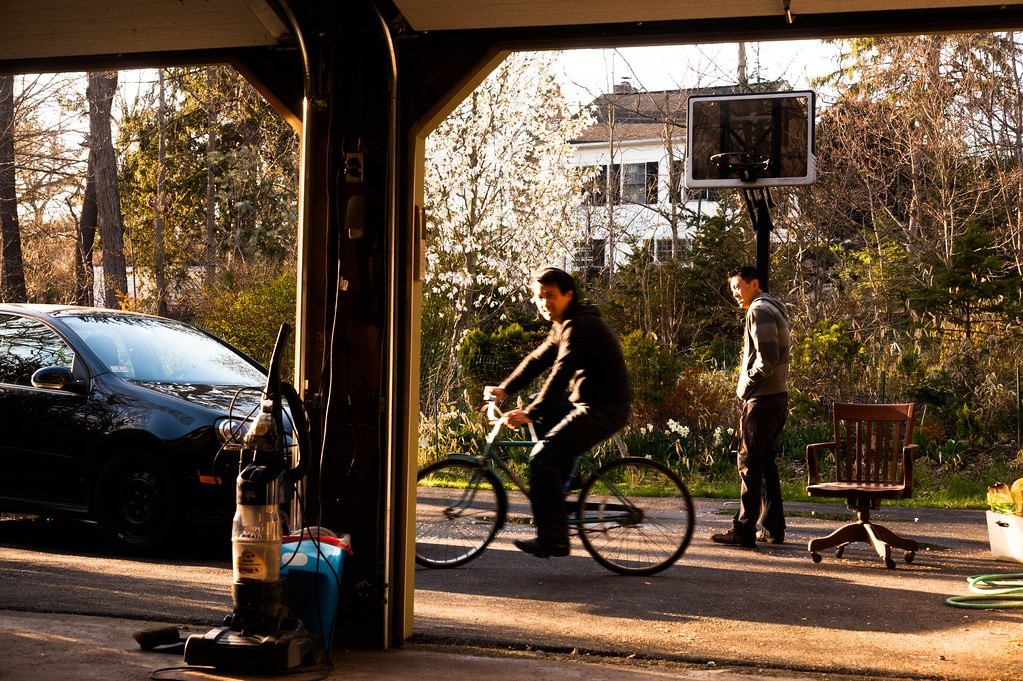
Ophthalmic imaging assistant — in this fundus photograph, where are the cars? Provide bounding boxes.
[0,301,301,557]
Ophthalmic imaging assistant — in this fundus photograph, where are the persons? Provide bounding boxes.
[483,267,631,559]
[712,266,790,547]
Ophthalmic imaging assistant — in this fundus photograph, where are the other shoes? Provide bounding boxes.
[567,472,584,489]
[515,538,570,556]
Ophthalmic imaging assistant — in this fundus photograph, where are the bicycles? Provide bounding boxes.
[415,390,695,576]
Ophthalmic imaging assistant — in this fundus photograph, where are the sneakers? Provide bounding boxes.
[712,528,756,547]
[756,530,785,543]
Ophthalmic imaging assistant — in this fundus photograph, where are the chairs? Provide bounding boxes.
[805,401,920,567]
